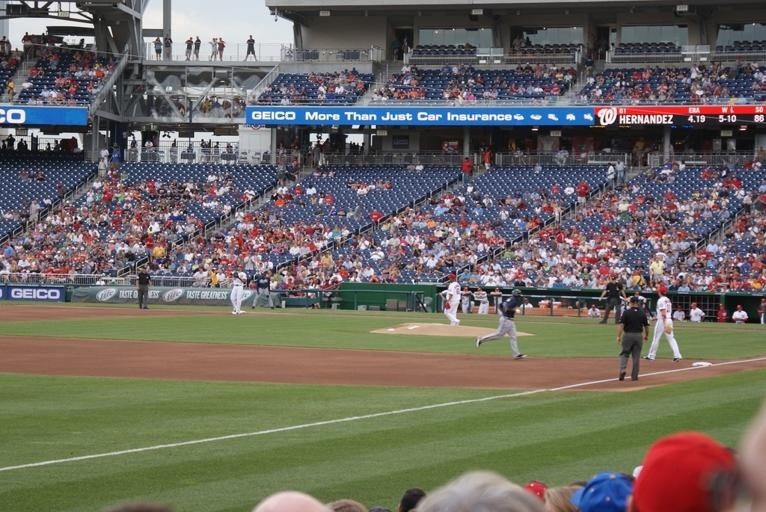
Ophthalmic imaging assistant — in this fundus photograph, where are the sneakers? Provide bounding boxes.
[475,338,482,348]
[616,319,620,323]
[620,371,626,379]
[632,375,638,380]
[673,358,679,362]
[600,319,607,323]
[515,352,527,359]
[642,356,648,358]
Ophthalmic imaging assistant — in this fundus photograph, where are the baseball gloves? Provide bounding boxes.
[444,303,450,308]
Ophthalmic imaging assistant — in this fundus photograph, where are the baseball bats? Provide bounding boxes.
[644,303,654,319]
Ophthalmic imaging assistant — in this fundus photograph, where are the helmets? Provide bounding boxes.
[447,274,455,280]
[511,288,522,295]
[611,274,618,278]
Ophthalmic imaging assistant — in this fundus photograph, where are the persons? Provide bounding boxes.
[229,264,247,313]
[237,133,766,289]
[617,296,649,381]
[259,61,766,106]
[458,286,503,313]
[521,297,766,325]
[252,272,275,310]
[443,274,461,326]
[136,266,152,309]
[597,272,626,324]
[267,277,340,309]
[477,289,529,359]
[152,36,258,61]
[1,31,111,109]
[113,402,766,511]
[0,130,257,284]
[642,285,682,364]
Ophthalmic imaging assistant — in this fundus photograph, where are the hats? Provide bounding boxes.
[657,288,666,294]
[691,303,697,306]
[523,481,548,502]
[678,305,681,308]
[633,432,736,512]
[630,297,639,304]
[719,304,724,307]
[571,471,635,512]
